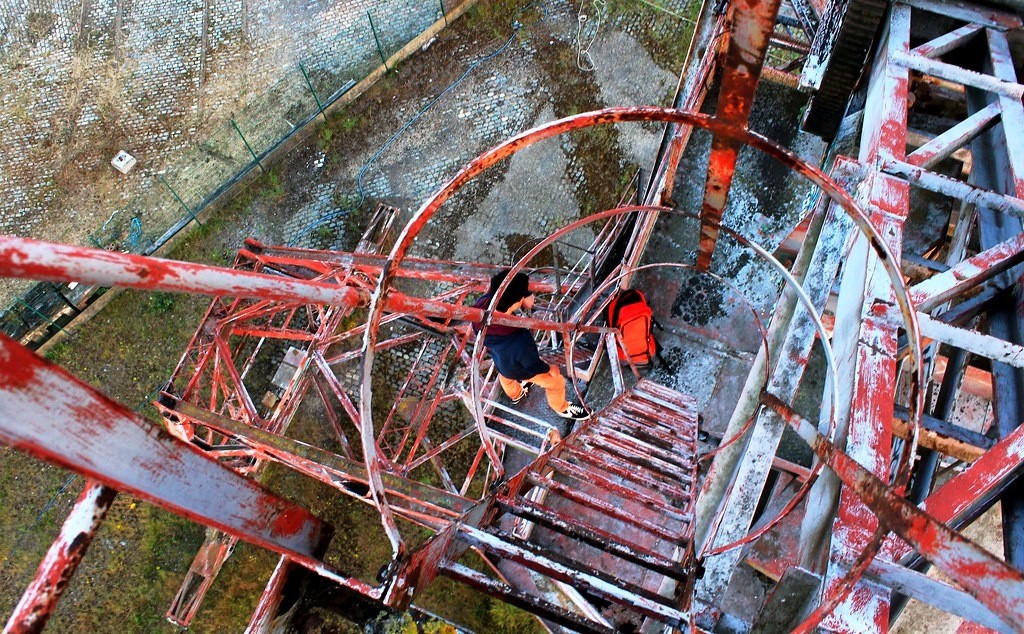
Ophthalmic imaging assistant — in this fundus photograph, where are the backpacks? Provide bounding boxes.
[605,288,662,370]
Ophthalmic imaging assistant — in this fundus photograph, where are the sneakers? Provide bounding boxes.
[557,403,595,421]
[510,379,535,405]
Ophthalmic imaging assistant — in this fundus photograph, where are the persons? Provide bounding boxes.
[462,266,595,425]
